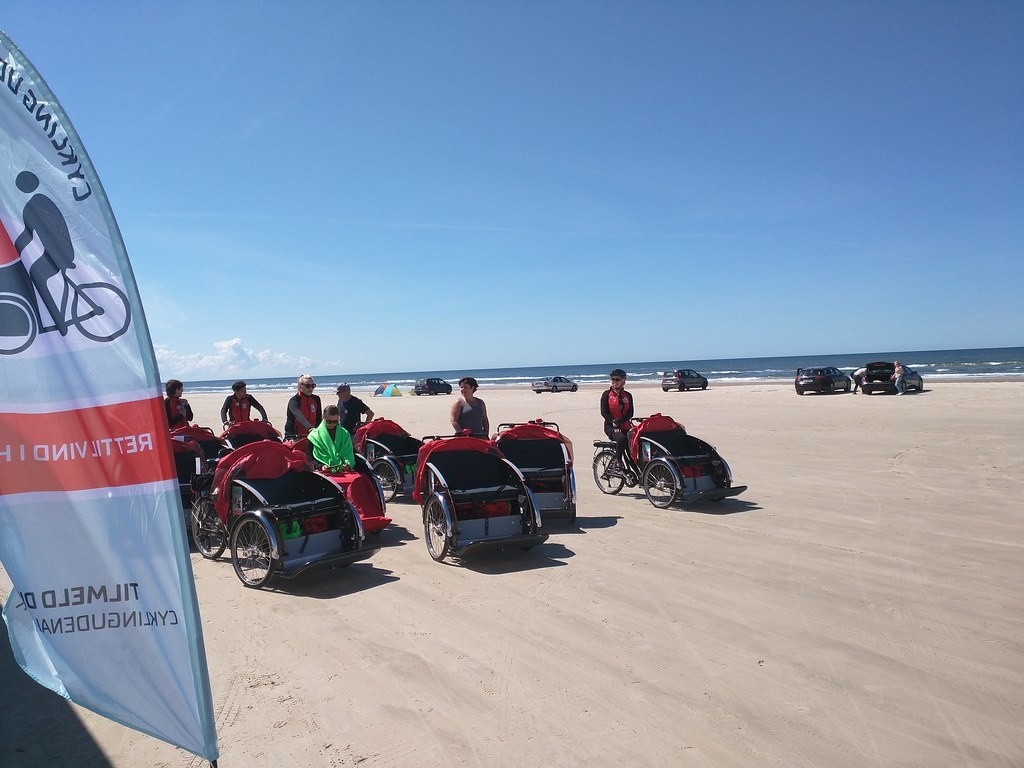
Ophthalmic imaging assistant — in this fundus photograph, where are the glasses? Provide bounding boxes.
[301,383,316,388]
[611,378,622,382]
[325,418,338,424]
[179,388,184,392]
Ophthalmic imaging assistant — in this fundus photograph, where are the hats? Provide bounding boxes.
[333,383,350,392]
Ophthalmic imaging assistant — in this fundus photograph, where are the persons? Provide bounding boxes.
[220,381,268,434]
[852,368,868,394]
[335,381,374,454]
[285,374,323,445]
[600,369,633,473]
[450,377,490,440]
[305,405,355,473]
[164,379,193,431]
[889,361,905,396]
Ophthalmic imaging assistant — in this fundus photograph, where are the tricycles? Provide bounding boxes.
[487,419,577,524]
[281,430,392,534]
[190,445,381,591]
[216,421,284,454]
[167,423,228,537]
[589,413,749,507]
[349,416,427,505]
[411,430,546,565]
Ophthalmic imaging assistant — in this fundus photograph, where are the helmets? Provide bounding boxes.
[610,369,627,380]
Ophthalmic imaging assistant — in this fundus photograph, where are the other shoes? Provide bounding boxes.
[615,460,621,471]
[853,392,857,395]
[862,392,865,394]
[896,391,905,396]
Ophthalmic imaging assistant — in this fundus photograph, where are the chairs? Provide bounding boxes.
[177,423,727,525]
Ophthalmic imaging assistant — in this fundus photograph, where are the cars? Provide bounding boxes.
[858,360,925,393]
[414,377,454,397]
[795,366,853,394]
[662,368,708,392]
[531,375,579,394]
